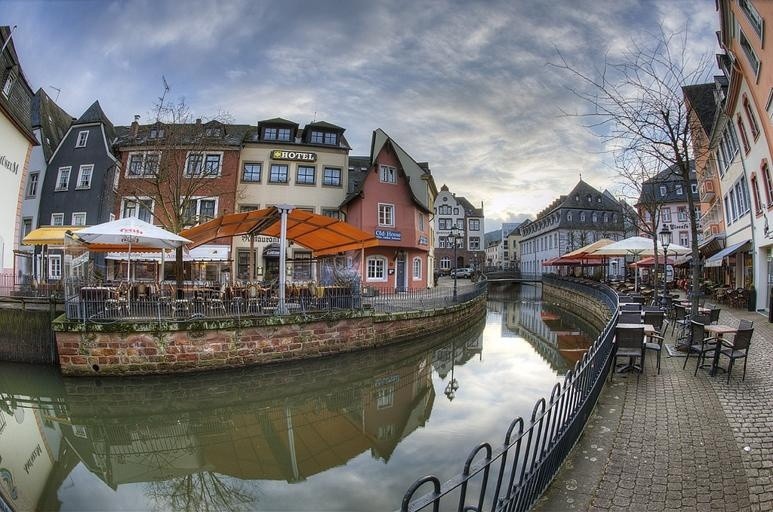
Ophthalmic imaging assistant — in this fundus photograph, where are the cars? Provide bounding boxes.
[450,267,474,279]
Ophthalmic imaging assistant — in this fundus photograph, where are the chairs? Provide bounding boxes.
[102,279,133,318]
[609,283,754,384]
[154,279,323,320]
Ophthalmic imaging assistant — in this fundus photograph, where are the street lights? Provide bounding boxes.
[446,224,462,302]
[658,224,671,310]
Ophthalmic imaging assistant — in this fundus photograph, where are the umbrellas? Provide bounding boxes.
[540,236,688,295]
[76,216,192,311]
